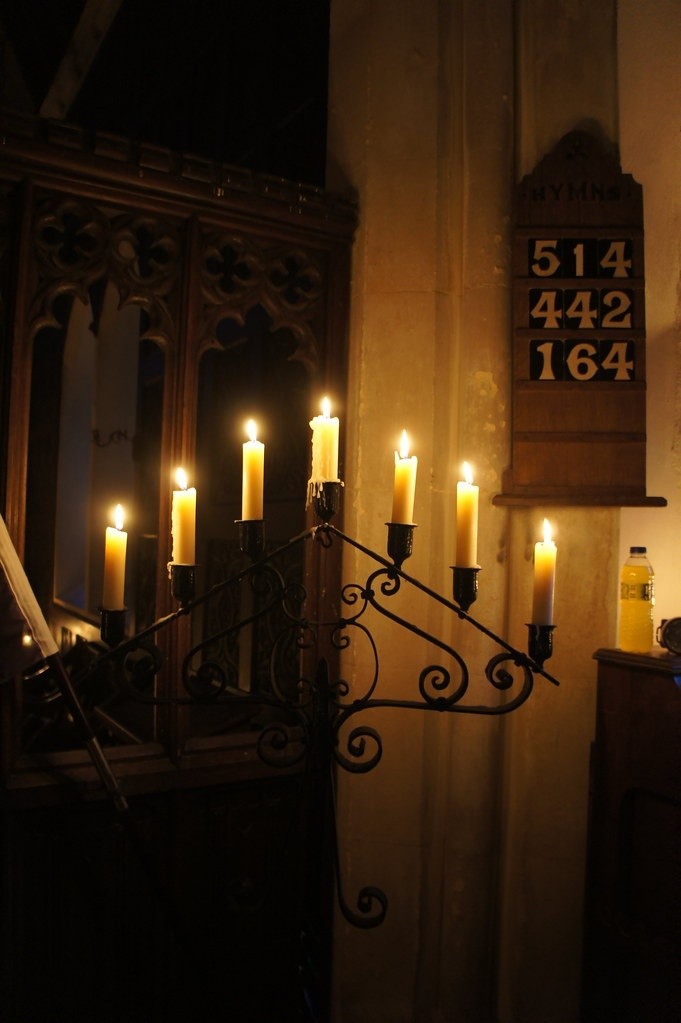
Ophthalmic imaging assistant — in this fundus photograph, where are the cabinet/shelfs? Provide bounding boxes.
[582,652,681,1023]
[0,103,360,1023]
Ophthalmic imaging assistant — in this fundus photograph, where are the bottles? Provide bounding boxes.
[617,545,655,655]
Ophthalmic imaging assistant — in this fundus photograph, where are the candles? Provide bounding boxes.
[457,463,479,567]
[172,469,196,565]
[309,396,339,481]
[531,519,557,625]
[390,431,417,523]
[105,503,129,611]
[241,419,263,519]
